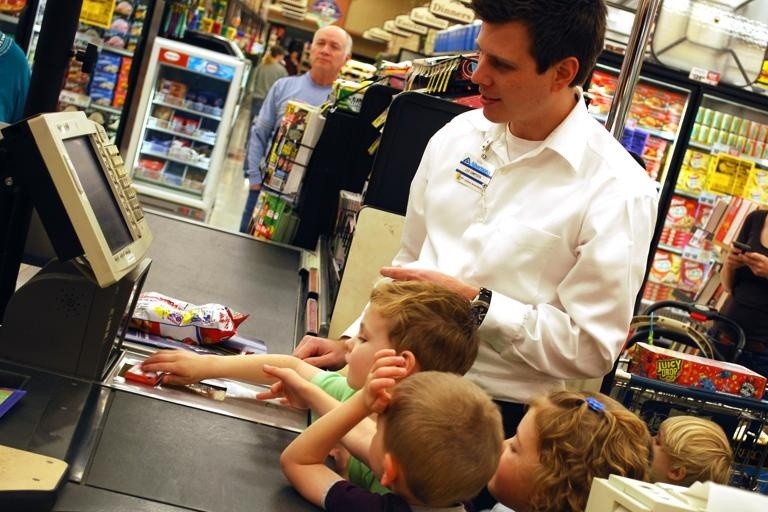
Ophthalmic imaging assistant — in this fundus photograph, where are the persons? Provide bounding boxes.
[237,24,354,234]
[243,44,291,159]
[255,347,507,511]
[0,29,35,125]
[651,415,734,489]
[699,209,768,442]
[290,1,660,511]
[140,277,482,498]
[486,387,651,512]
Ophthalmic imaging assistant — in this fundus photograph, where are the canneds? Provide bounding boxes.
[690,106,768,161]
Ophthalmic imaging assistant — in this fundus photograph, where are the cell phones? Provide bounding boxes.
[730,240,752,255]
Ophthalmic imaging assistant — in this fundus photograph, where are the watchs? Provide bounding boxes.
[469,289,492,328]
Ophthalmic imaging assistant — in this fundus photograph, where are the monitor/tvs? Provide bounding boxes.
[3,255,153,381]
[0,110,157,284]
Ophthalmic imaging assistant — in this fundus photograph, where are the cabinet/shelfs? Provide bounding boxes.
[632,87,768,352]
[0,0,265,150]
[583,59,690,196]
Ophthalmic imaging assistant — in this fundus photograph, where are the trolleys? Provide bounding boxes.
[599,301,768,490]
